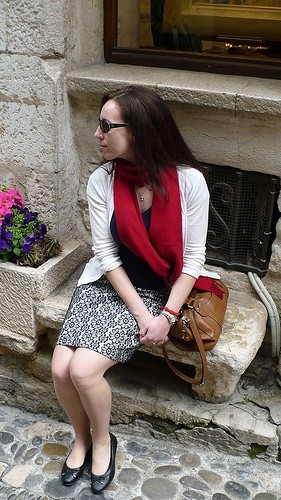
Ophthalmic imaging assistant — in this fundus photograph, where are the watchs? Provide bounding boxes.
[161,310,176,324]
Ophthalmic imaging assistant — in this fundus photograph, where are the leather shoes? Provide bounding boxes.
[61,443,92,486]
[91,432,117,494]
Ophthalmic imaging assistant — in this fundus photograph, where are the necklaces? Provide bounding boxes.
[137,187,146,201]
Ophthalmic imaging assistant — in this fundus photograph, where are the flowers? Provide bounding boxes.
[0,184,49,264]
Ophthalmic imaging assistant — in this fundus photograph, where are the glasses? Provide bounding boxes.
[98,118,129,133]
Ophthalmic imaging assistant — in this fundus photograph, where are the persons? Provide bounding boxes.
[51,86,210,493]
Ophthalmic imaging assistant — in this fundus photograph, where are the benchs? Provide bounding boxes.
[36,262,268,403]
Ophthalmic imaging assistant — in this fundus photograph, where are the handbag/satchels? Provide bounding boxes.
[167,279,229,352]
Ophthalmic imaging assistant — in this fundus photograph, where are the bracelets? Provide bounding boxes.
[164,307,180,317]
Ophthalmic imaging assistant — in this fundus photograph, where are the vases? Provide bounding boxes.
[0,239,84,339]
